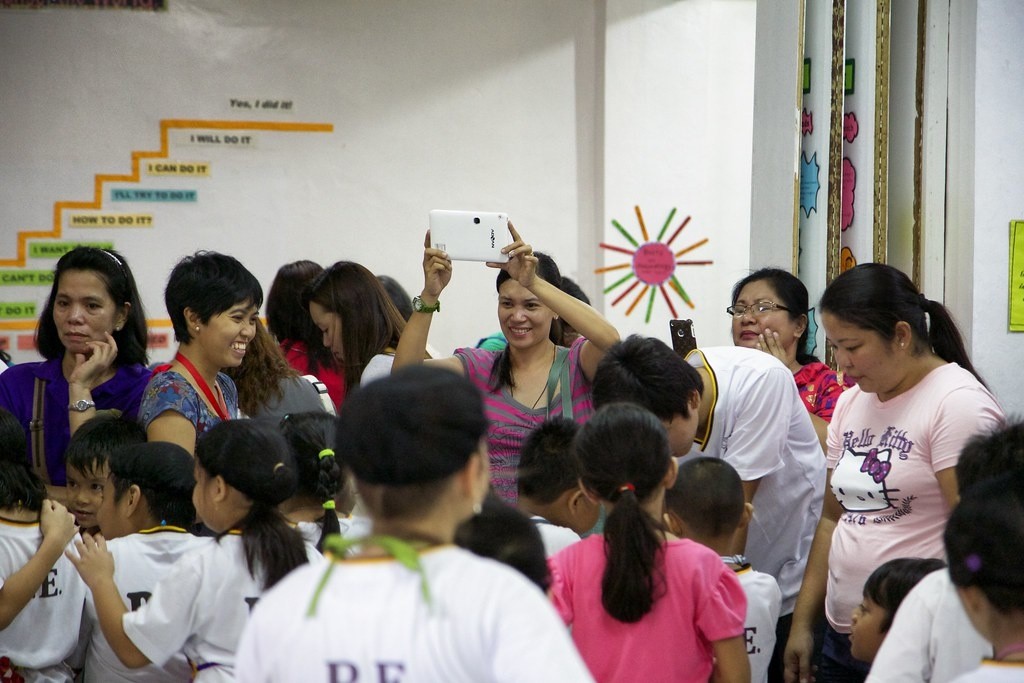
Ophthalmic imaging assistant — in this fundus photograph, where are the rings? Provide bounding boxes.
[525,252,534,257]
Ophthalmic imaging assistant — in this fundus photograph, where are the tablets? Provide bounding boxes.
[429,209,511,262]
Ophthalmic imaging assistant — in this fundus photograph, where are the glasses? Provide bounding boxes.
[727,302,801,319]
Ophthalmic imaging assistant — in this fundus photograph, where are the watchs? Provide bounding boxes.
[412,295,441,313]
[68,399,95,411]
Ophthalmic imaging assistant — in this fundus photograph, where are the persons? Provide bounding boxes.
[228,360,595,682]
[724,268,857,462]
[389,206,620,507]
[472,274,592,352]
[133,249,264,468]
[0,400,1024,683]
[783,264,1011,682]
[589,334,830,683]
[0,247,156,532]
[216,259,432,430]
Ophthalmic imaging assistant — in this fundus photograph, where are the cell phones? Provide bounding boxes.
[670,319,698,357]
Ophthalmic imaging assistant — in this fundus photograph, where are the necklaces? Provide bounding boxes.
[369,527,447,549]
[994,641,1024,660]
[529,518,550,525]
[174,350,231,423]
[505,344,556,408]
